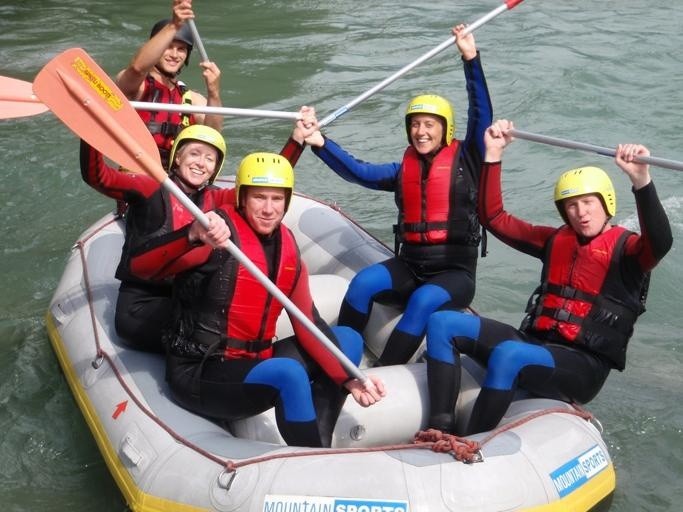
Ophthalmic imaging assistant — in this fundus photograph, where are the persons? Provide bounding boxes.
[113,0,223,224]
[296,23,494,370]
[124,150,388,448]
[424,118,674,440]
[78,104,321,357]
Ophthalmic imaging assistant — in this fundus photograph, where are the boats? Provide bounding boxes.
[43,174,615,511]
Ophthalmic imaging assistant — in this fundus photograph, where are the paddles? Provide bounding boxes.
[0,76,305,121]
[32,47,381,399]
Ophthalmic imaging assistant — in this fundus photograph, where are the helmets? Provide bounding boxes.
[405,95,455,146]
[169,125,227,183]
[150,19,194,66]
[555,166,616,226]
[234,152,294,214]
